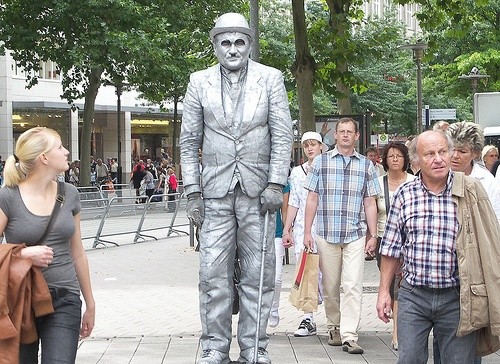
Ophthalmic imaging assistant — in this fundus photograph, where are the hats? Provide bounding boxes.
[301,131,330,152]
[482,145,497,166]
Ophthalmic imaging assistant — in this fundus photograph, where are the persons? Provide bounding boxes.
[180,12,294,364]
[268,117,382,354]
[0,127,95,364]
[57,156,118,192]
[130,148,178,213]
[369,120,500,364]
[0,155,19,186]
[364,146,385,261]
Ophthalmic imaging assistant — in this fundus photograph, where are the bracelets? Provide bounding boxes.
[282,233,289,236]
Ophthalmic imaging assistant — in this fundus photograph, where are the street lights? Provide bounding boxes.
[105,75,134,202]
[457,66,490,95]
[396,42,429,136]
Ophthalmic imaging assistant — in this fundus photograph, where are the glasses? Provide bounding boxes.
[387,153,405,160]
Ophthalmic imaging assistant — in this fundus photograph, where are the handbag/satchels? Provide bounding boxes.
[288,247,320,313]
[105,180,114,189]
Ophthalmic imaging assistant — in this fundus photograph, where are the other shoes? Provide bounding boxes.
[270,310,279,327]
[392,340,398,350]
[365,253,373,261]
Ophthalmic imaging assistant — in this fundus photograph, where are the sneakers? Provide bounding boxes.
[328,327,342,345]
[342,340,364,354]
[294,318,316,336]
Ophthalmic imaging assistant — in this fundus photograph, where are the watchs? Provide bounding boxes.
[371,235,378,238]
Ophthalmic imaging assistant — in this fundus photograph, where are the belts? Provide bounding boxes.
[49,288,67,297]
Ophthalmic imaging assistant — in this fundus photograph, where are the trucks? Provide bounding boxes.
[472,91,500,159]
